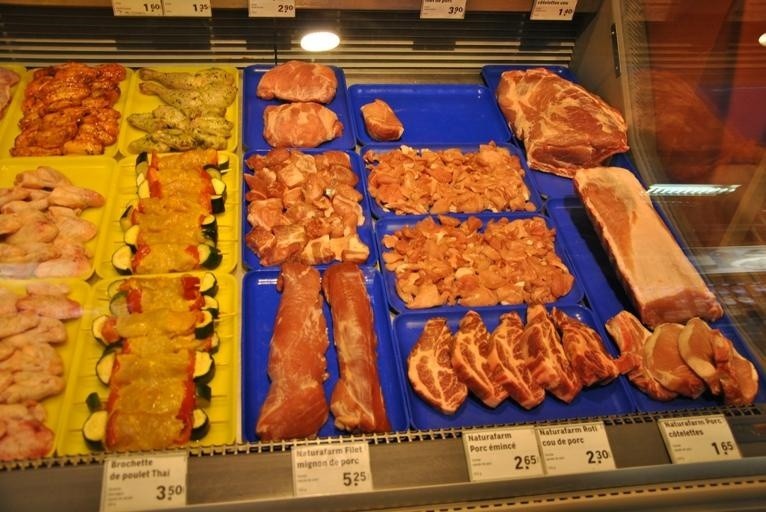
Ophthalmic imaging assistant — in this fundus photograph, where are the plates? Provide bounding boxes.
[241,267,410,442]
[546,196,762,412]
[118,65,239,153]
[481,65,649,206]
[0,278,91,462]
[94,150,240,272]
[241,152,380,271]
[359,144,544,214]
[0,156,116,281]
[57,273,240,457]
[375,212,583,312]
[392,303,638,430]
[1,67,135,159]
[348,82,512,147]
[242,63,356,152]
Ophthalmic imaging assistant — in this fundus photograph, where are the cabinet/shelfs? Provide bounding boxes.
[0,1,766,512]
